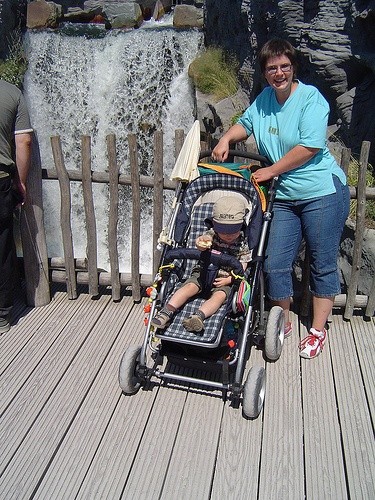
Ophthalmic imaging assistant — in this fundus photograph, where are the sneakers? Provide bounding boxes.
[299,328,327,359]
[278,322,293,338]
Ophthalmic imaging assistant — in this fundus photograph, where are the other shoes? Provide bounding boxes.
[0,313,10,334]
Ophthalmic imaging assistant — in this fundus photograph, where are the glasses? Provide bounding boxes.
[265,63,292,74]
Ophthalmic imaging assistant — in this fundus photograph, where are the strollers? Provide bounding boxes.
[116,148,286,419]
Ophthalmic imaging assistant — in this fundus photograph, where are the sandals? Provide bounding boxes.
[151,307,174,329]
[183,315,204,332]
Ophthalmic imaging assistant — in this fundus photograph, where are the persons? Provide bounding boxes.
[150,196,252,332]
[0,79,34,332]
[211,37,351,359]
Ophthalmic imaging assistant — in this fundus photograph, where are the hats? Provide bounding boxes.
[212,196,245,234]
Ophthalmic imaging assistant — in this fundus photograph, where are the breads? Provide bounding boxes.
[199,239,212,248]
